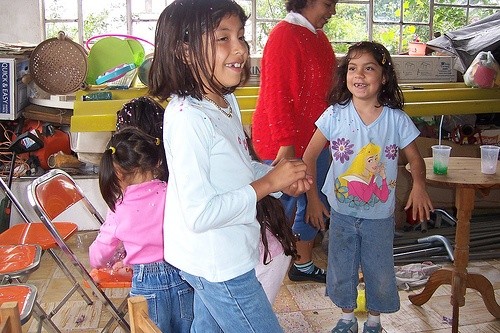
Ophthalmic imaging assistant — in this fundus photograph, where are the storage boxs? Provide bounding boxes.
[0,58,30,120]
[382,55,457,83]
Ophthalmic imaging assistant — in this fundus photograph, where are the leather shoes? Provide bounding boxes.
[287,265,326,283]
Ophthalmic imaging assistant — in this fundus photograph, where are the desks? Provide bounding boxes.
[406,156,500,333]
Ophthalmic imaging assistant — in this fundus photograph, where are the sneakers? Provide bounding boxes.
[361,323,382,333]
[329,318,358,333]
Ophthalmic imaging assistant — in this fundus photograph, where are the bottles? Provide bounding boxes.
[81,91,112,101]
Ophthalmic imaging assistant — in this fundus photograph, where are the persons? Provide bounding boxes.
[251,0,339,283]
[148,0,314,333]
[303,41,434,333]
[89,41,300,333]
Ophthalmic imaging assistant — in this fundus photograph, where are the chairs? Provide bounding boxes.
[0,243,59,333]
[0,285,56,333]
[0,177,93,333]
[26,169,132,333]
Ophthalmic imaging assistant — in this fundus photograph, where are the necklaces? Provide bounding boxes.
[201,93,232,118]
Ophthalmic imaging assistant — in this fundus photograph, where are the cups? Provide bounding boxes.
[431,145,452,175]
[480,145,500,174]
